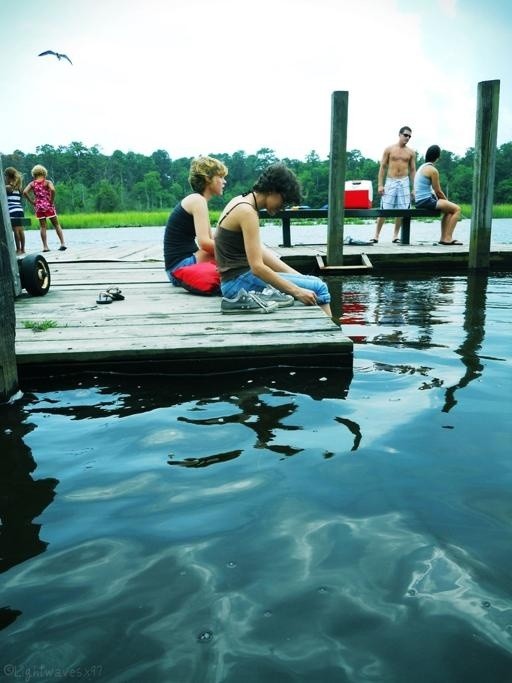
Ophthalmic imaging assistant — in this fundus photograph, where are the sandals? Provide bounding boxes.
[96,287,124,304]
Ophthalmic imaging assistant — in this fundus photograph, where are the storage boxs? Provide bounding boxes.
[343,180,375,207]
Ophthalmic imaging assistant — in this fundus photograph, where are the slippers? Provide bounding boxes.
[392,239,401,243]
[370,239,378,243]
[439,240,463,245]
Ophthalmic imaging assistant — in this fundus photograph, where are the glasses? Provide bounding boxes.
[404,134,411,139]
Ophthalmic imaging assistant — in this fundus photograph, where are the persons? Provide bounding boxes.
[370,280,406,349]
[366,125,417,244]
[23,164,68,252]
[213,166,334,318]
[163,155,229,287]
[414,145,464,245]
[4,166,25,254]
[406,277,442,349]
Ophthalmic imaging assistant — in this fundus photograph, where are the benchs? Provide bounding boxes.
[255,205,443,247]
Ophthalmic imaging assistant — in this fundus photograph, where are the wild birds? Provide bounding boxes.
[38,51,72,64]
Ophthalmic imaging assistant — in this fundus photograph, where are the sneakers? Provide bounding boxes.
[221,288,278,314]
[249,284,294,308]
